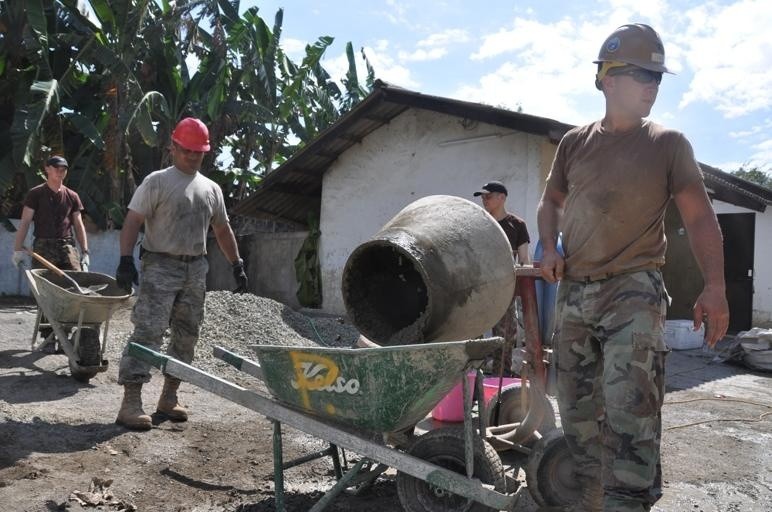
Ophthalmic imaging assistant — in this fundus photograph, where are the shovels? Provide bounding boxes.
[23,244,108,295]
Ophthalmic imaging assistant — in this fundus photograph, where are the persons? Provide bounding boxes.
[116,118,249,430]
[473,183,530,378]
[11,155,91,354]
[538,24,730,512]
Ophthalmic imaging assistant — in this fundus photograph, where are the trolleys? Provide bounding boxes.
[126,327,536,511]
[16,259,135,382]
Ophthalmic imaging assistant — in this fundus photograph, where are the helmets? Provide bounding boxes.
[171,117,211,153]
[592,20,678,75]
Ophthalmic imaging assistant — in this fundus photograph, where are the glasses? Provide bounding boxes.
[603,68,666,85]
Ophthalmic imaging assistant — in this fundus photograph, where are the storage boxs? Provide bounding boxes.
[664,319,706,351]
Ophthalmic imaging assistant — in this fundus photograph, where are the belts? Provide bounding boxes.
[567,262,669,283]
[144,246,208,264]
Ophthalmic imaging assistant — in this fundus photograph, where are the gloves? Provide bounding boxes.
[78,251,90,270]
[231,258,250,296]
[115,256,139,294]
[11,250,26,270]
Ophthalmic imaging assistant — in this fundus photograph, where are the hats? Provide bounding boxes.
[473,178,509,198]
[46,154,69,170]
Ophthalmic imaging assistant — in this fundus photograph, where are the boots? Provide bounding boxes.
[535,484,606,511]
[43,333,72,354]
[156,377,190,421]
[114,381,154,429]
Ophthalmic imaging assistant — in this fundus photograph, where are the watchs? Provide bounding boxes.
[82,249,91,254]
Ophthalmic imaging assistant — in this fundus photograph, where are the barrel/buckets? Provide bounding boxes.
[431,371,477,423]
[481,377,530,402]
